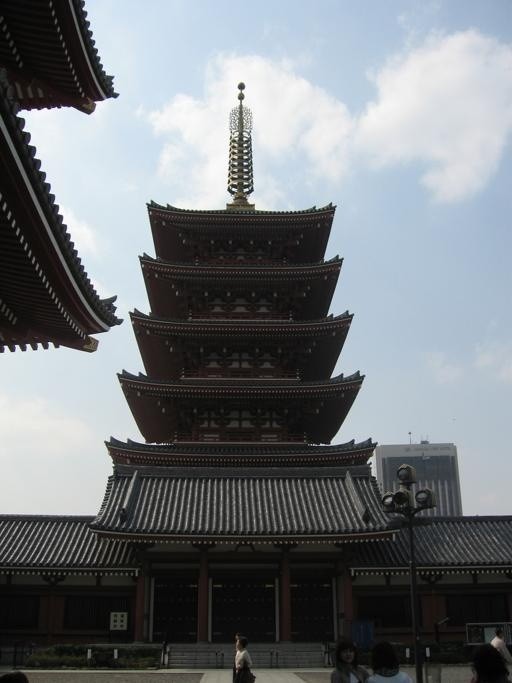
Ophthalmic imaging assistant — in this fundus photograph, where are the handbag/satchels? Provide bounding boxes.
[236,660,256,682]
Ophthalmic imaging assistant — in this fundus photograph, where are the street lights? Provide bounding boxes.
[381,460,436,681]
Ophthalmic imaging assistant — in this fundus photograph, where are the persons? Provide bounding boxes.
[467,643,511,683]
[361,640,413,682]
[329,637,370,682]
[489,628,511,663]
[233,635,256,683]
